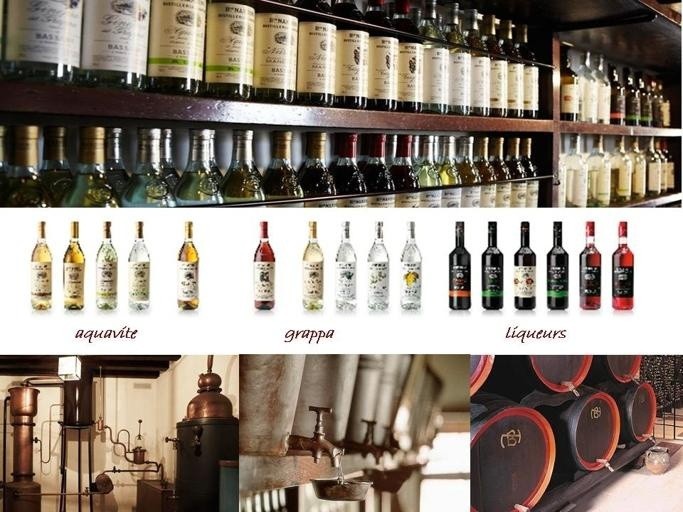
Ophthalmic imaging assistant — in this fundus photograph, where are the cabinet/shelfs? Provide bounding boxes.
[1,1,680,208]
[472,377,657,512]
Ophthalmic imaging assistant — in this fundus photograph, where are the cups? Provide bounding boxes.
[644,446,671,474]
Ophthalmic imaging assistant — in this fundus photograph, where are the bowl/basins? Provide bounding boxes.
[307,479,373,501]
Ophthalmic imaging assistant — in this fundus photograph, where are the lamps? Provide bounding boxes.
[56,354,83,382]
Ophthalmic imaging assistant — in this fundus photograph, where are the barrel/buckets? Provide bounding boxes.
[471,354,657,512]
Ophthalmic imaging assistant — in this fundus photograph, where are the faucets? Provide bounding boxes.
[358,417,384,465]
[383,425,402,450]
[288,406,346,468]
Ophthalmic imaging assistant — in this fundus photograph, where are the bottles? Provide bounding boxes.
[302,220,422,310]
[177,222,200,312]
[94,218,120,313]
[30,220,53,313]
[448,220,636,312]
[0,1,543,119]
[126,220,151,314]
[59,220,87,313]
[0,119,539,208]
[252,219,276,311]
[559,48,675,209]
